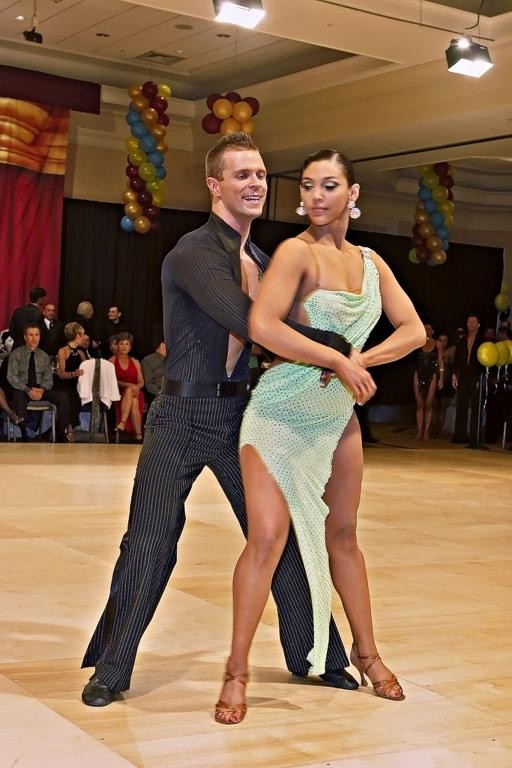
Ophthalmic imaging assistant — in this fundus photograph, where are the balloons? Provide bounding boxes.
[495,294,509,313]
[406,161,457,268]
[200,89,260,141]
[116,80,173,233]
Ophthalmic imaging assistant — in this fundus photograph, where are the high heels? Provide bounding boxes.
[9,415,25,425]
[350,639,408,703]
[114,419,127,433]
[133,432,144,443]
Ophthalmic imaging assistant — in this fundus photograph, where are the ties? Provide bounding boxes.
[27,351,38,389]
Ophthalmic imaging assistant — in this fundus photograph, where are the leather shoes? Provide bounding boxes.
[81,672,117,707]
[292,662,359,692]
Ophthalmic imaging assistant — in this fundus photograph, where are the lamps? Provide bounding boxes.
[445,0,494,78]
[211,0,266,29]
[23,0,43,44]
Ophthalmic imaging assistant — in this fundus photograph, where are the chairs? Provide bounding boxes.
[3,400,147,444]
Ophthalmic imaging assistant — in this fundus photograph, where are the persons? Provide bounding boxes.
[79,131,362,706]
[358,316,512,446]
[213,145,428,725]
[1,286,167,445]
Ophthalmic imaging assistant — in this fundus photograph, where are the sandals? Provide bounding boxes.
[214,654,247,724]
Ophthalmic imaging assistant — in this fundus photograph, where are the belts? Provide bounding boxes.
[160,375,254,399]
